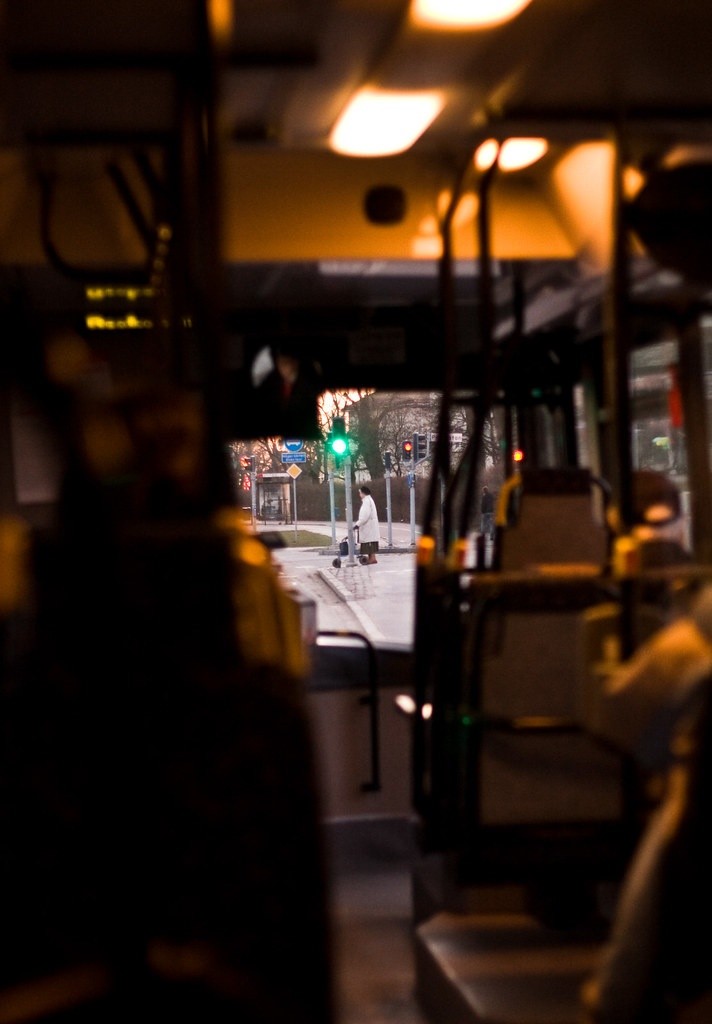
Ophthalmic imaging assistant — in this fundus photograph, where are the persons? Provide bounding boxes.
[480,485,494,540]
[354,485,379,565]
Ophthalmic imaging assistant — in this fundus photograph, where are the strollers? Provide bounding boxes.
[331,526,368,568]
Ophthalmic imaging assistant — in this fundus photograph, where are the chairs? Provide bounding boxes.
[451,464,617,837]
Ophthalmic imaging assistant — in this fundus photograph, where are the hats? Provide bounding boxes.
[361,486,371,495]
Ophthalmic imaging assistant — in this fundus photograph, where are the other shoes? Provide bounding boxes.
[367,558,377,564]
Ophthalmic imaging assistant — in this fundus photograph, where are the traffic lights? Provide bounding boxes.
[331,417,349,457]
[402,440,412,462]
[244,455,255,473]
[414,431,428,463]
